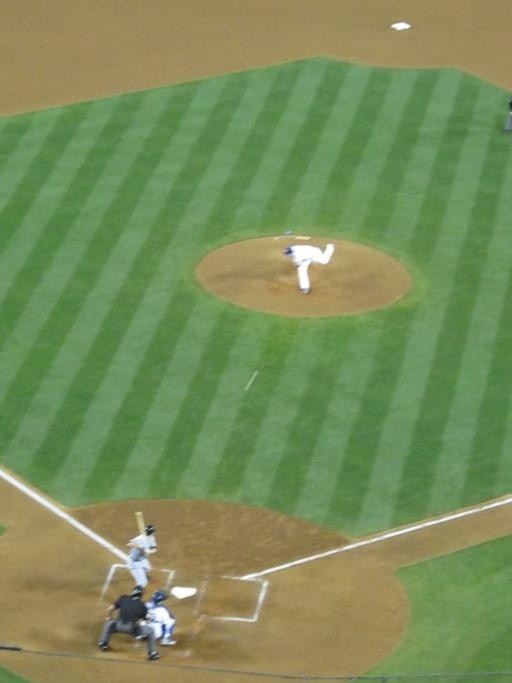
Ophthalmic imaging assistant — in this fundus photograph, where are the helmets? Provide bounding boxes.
[151,591,168,607]
[145,524,156,536]
[130,585,144,598]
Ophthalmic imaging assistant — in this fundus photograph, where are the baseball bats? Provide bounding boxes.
[135,511,145,537]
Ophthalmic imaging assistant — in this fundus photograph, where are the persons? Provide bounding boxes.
[96,585,164,660]
[504,96,512,131]
[126,524,159,589]
[136,591,177,646]
[282,243,334,295]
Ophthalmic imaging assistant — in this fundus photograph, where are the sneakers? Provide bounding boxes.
[161,639,177,646]
[135,634,147,640]
[148,651,161,660]
[97,641,109,651]
